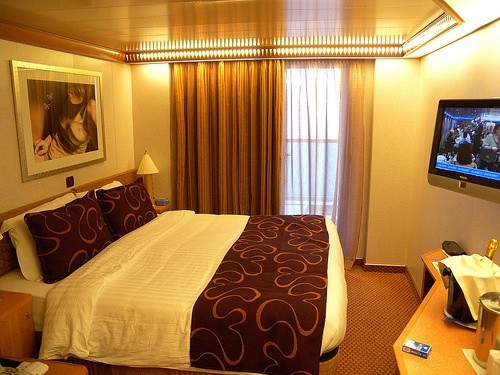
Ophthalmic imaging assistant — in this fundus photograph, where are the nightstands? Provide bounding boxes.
[153,204,171,213]
[0,291,36,358]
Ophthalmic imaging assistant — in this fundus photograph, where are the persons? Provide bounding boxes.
[34,83,99,162]
[445,115,500,173]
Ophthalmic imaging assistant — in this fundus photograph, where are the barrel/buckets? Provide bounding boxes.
[473,290,500,369]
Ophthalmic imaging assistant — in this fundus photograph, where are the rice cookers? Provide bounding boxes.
[442,268,478,323]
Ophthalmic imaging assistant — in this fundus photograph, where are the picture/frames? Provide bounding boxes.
[10,60,107,182]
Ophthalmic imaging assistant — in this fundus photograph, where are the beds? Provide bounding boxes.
[0,167,348,375]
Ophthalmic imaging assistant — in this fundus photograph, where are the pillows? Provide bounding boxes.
[96,176,158,240]
[24,188,116,285]
[77,180,123,199]
[0,192,77,281]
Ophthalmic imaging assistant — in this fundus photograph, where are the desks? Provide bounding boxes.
[393,249,486,375]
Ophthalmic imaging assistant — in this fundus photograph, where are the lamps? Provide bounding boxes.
[137,150,160,205]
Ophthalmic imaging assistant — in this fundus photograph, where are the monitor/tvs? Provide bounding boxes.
[427,95,500,204]
